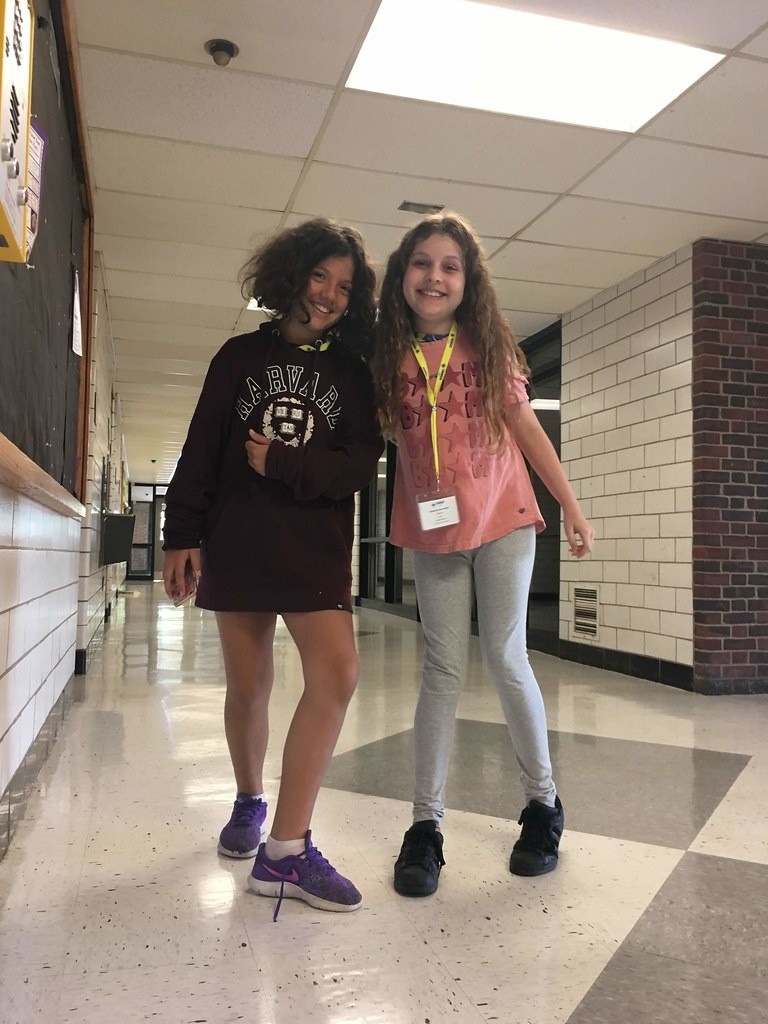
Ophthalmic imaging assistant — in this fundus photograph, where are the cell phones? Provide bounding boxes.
[172,558,197,607]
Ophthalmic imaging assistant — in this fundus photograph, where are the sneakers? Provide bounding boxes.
[509,794,564,876]
[217,792,268,859]
[247,830,362,922]
[393,820,446,897]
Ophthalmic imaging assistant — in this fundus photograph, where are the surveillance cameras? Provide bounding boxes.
[211,41,234,66]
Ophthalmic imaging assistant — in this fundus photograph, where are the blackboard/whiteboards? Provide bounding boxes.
[0,0,97,524]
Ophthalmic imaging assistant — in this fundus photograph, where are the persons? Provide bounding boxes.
[367,209,593,899]
[161,216,385,913]
